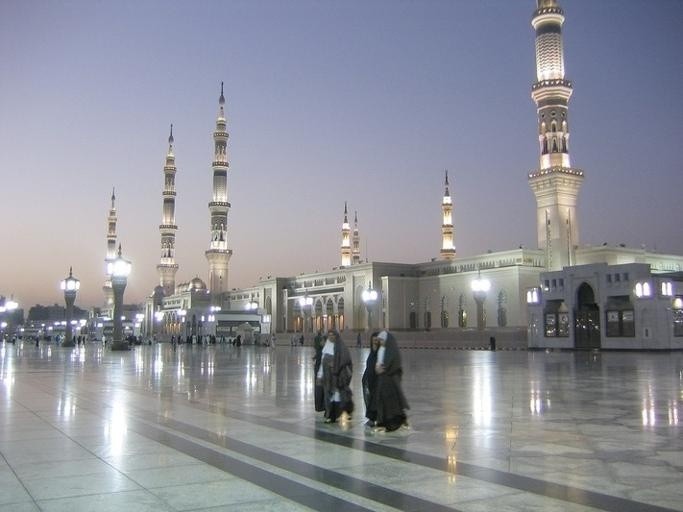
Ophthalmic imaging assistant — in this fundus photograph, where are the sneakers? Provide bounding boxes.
[324,417,335,423]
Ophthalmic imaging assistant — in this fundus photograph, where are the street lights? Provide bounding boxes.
[470,266,492,333]
[61,266,80,347]
[362,282,378,330]
[5,292,18,341]
[107,244,133,350]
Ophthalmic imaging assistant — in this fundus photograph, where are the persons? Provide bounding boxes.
[124,334,158,345]
[171,335,177,351]
[299,336,304,346]
[101,335,106,345]
[312,327,410,433]
[270,333,276,349]
[178,333,241,347]
[489,336,496,350]
[12,332,86,347]
